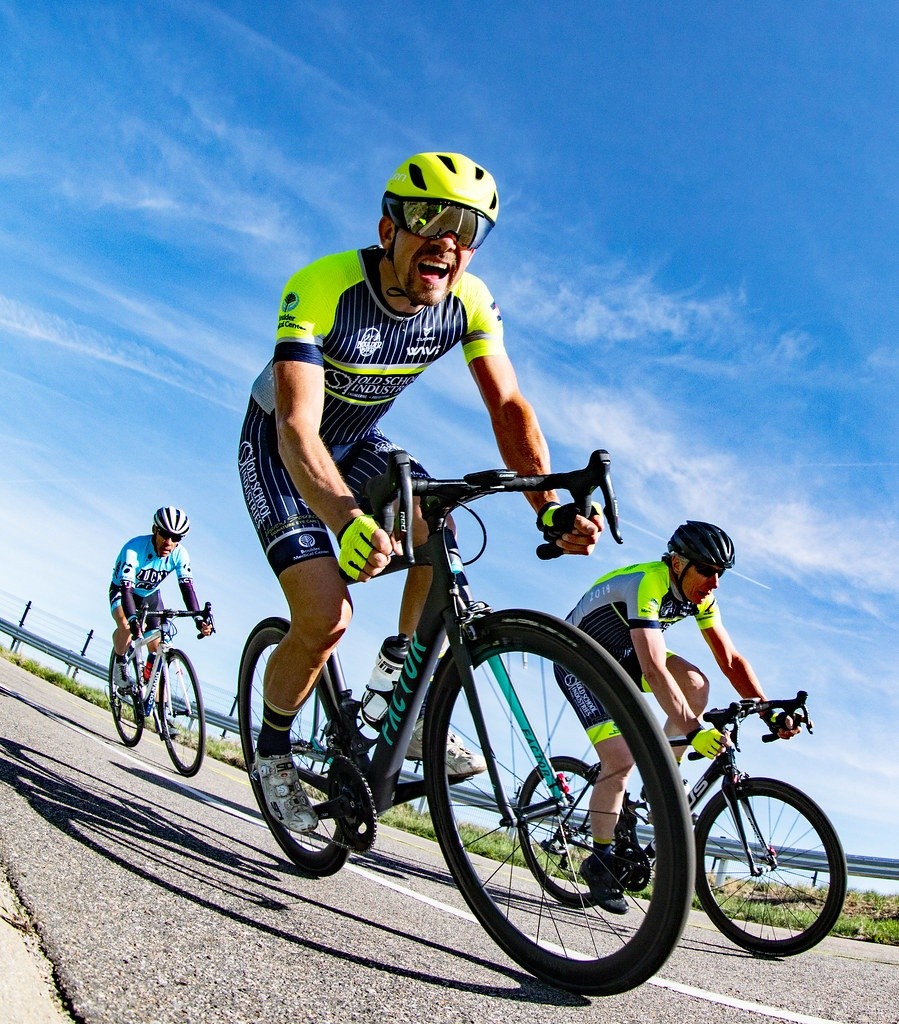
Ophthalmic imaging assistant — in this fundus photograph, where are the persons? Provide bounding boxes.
[237,151,606,832]
[554,520,814,915]
[109,506,212,735]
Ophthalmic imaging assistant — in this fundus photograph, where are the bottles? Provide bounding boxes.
[139,659,146,688]
[143,652,156,681]
[360,633,409,732]
[647,779,688,826]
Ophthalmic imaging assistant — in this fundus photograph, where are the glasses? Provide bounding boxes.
[155,526,181,543]
[694,561,727,580]
[396,198,492,249]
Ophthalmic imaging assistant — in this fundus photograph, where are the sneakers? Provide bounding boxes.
[405,718,487,776]
[255,749,318,832]
[164,722,179,738]
[580,857,628,914]
[114,655,129,690]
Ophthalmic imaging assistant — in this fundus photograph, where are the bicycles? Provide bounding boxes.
[103,600,216,778]
[507,687,850,959]
[234,446,700,998]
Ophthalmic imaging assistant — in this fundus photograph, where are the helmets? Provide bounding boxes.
[670,521,736,568]
[153,504,190,537]
[385,151,498,239]
